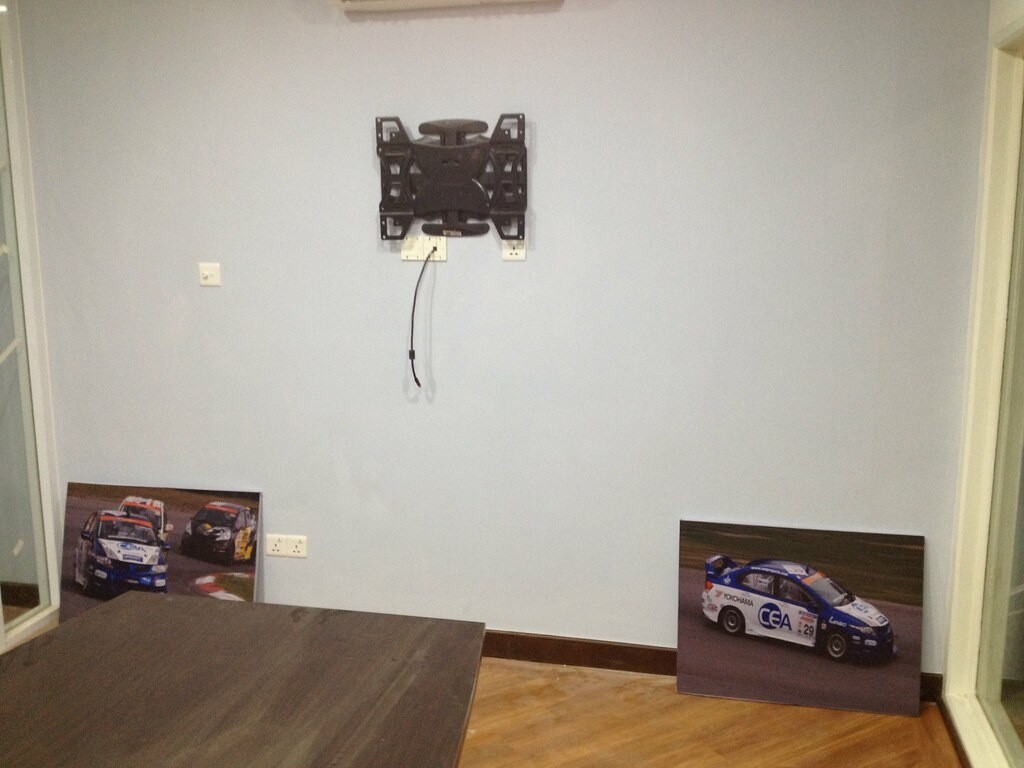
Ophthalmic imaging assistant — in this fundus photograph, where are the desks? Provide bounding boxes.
[0,592,489,768]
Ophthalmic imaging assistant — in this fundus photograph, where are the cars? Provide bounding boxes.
[178,503,257,564]
[118,495,173,546]
[702,554,898,666]
[71,512,169,598]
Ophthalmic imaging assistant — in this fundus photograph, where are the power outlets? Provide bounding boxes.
[286,536,308,558]
[503,239,525,261]
[266,534,285,557]
